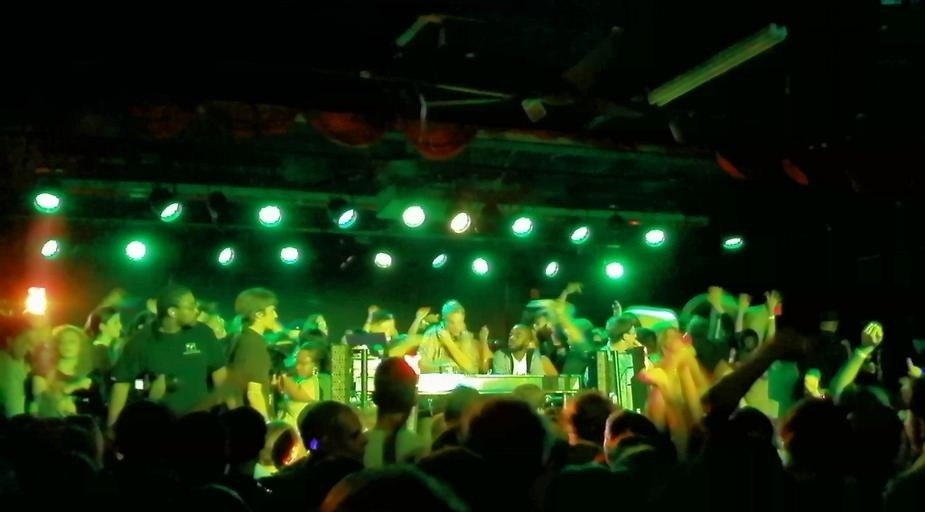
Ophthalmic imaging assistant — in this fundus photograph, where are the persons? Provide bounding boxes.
[0,279,924,511]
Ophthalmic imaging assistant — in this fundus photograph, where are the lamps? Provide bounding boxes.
[648,22,787,108]
[145,178,185,223]
[325,196,358,229]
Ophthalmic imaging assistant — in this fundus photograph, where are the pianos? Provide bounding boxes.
[418,371,581,395]
